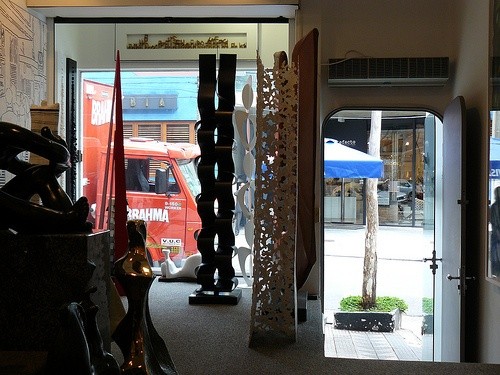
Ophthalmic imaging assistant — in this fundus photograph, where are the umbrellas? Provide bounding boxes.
[489,135,500,180]
[324,143,385,187]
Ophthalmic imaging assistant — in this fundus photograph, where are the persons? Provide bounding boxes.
[489,186,500,277]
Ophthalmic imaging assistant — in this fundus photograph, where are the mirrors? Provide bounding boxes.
[484,107,500,287]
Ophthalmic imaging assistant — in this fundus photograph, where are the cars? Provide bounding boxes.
[332,179,433,207]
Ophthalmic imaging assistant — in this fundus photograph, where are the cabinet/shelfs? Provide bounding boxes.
[0,228,111,352]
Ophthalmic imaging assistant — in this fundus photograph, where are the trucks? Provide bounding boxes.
[81,78,241,268]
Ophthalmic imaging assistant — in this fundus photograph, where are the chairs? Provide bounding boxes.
[133,168,151,192]
